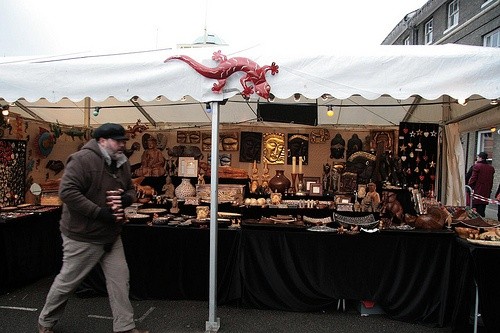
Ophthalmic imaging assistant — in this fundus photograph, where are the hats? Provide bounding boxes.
[94,123,130,141]
[478,152,488,159]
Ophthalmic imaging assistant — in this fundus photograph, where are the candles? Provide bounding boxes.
[292,156,296,173]
[298,156,303,174]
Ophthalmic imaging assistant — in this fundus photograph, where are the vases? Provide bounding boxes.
[269,170,291,195]
[174,179,195,201]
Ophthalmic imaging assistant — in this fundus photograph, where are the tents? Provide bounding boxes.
[1,37,500,206]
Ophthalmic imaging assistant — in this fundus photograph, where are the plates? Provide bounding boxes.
[128,214,151,223]
[467,238,500,246]
[1,207,17,211]
[191,218,231,227]
[177,157,198,177]
[209,212,243,218]
[138,207,167,216]
[452,220,500,228]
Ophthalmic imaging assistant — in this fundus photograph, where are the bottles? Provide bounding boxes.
[175,178,195,200]
[269,170,290,194]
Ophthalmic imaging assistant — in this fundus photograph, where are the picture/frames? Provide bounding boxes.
[303,177,323,196]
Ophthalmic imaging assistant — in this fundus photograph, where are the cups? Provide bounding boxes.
[196,206,209,219]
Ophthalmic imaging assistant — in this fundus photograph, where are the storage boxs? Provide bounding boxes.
[195,183,246,205]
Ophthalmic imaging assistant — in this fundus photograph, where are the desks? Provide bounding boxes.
[449,224,500,333]
[61,219,456,330]
[134,176,250,199]
[0,206,63,295]
[138,202,400,223]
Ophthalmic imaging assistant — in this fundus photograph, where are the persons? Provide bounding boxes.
[251,160,272,195]
[134,136,166,177]
[38,123,150,333]
[263,131,285,162]
[361,183,380,210]
[468,152,495,218]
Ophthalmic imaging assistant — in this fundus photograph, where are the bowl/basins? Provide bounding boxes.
[455,227,479,240]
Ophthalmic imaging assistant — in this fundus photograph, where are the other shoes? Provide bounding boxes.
[38,322,52,333]
[113,327,150,333]
